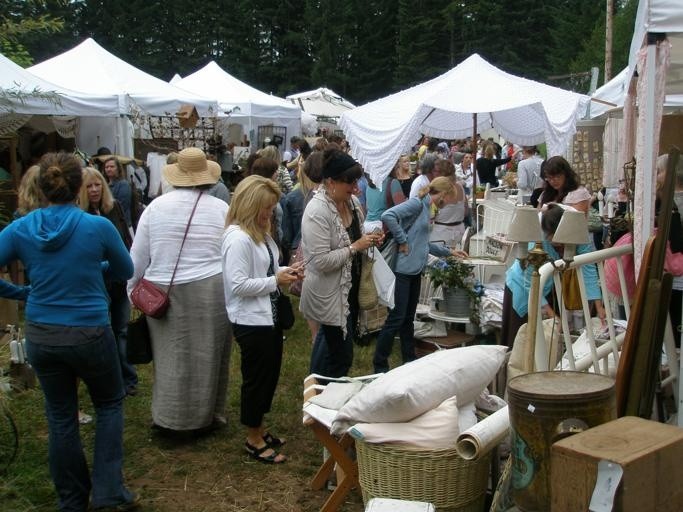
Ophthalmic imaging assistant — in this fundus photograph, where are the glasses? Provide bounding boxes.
[545,174,560,182]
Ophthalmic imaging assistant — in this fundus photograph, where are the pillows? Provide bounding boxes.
[330,342,510,424]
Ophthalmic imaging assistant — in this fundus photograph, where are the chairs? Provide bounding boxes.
[460,203,516,285]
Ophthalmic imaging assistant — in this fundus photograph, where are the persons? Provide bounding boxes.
[0,133,683,511]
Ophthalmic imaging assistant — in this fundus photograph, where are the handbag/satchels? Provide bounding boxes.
[127,279,169,364]
[358,255,378,310]
[381,238,399,273]
[277,242,304,329]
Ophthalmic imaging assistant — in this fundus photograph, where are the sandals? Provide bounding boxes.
[245,432,286,463]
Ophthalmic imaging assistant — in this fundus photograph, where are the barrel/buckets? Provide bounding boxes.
[508,371,619,511]
[442,286,470,318]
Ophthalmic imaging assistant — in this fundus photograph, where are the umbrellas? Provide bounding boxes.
[336,50,592,231]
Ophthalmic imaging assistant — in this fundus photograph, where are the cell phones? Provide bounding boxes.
[289,252,315,274]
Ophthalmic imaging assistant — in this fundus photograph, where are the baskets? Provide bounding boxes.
[354,409,492,512]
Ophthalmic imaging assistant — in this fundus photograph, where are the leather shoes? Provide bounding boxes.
[77,416,92,424]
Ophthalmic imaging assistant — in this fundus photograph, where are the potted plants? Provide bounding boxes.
[421,256,485,326]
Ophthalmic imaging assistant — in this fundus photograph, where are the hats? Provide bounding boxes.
[163,147,222,188]
[324,149,358,178]
